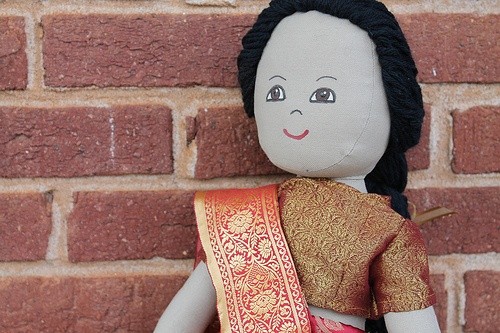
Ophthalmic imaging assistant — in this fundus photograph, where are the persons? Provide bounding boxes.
[152,1,443,332]
